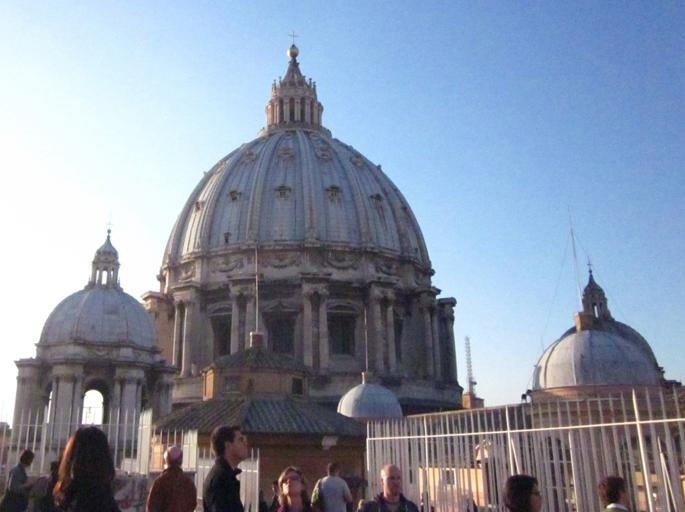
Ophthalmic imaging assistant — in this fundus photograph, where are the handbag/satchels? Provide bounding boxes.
[311,487,319,503]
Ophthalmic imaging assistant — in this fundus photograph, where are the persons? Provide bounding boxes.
[202,424,249,512]
[267,461,418,512]
[501,474,544,511]
[597,476,632,512]
[144,445,198,512]
[1,426,122,512]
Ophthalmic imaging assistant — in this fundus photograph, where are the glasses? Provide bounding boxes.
[283,476,301,483]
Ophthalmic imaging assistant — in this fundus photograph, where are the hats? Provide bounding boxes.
[164,447,182,464]
